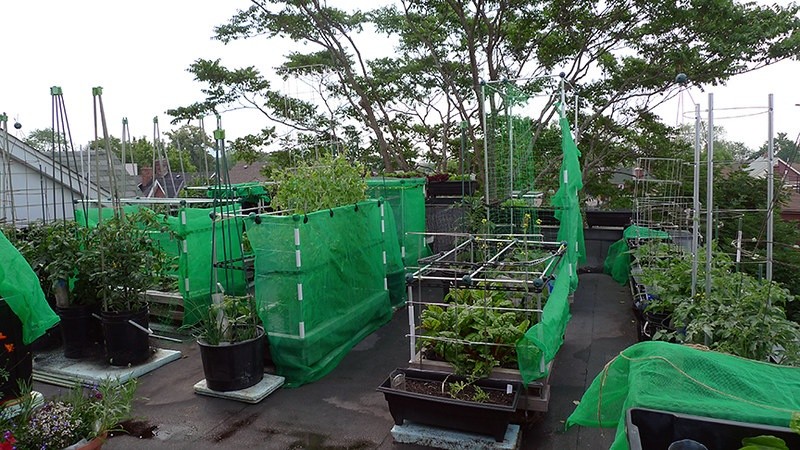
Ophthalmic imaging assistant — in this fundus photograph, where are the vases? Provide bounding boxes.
[75,427,108,450]
[626,404,800,450]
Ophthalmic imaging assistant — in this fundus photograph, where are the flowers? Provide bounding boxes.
[0,369,143,450]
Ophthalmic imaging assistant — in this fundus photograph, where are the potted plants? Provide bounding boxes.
[0,205,178,399]
[376,355,523,443]
[631,237,800,368]
[178,291,265,392]
